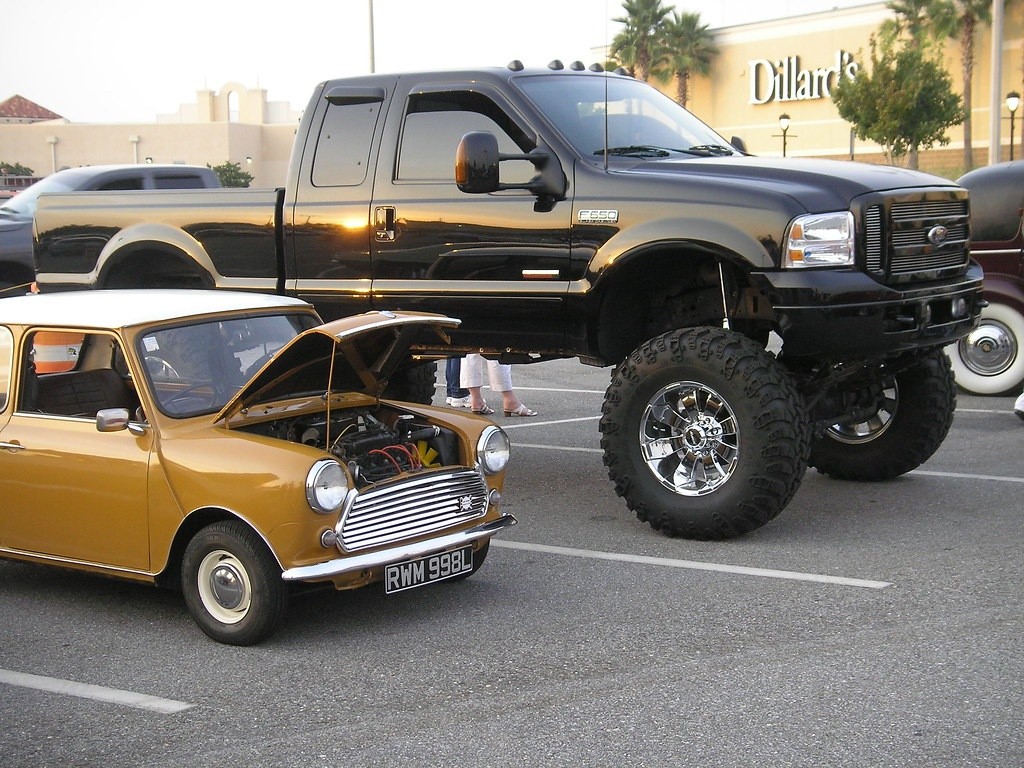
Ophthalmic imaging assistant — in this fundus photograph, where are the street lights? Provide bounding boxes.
[779,112,791,156]
[1003,92,1022,160]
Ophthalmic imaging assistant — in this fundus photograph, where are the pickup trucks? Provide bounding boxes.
[32,61,990,542]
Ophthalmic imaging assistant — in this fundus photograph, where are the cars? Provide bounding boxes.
[0,162,223,299]
[951,159,1023,399]
[0,287,523,647]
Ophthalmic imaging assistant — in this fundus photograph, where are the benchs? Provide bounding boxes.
[33,368,129,414]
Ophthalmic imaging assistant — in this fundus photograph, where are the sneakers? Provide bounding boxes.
[446,396,452,405]
[451,394,487,408]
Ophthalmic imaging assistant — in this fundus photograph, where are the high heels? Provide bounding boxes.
[504,403,538,417]
[471,403,494,415]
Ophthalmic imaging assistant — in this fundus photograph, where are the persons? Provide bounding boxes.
[445,357,486,408]
[460,353,538,416]
